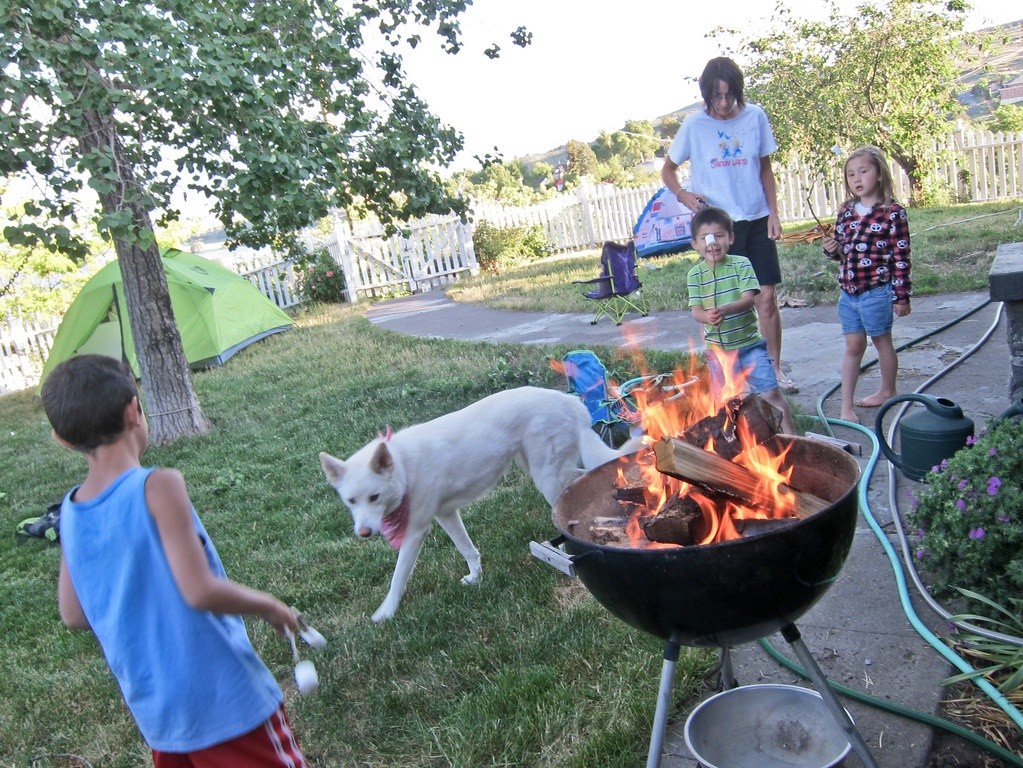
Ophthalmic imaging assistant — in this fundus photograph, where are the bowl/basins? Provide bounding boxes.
[683,683,855,768]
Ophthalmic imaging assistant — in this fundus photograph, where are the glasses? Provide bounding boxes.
[711,94,735,101]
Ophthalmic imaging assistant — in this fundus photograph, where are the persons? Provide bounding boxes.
[660,57,797,392]
[41,354,310,768]
[820,146,912,423]
[687,208,794,436]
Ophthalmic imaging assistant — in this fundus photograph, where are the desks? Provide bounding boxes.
[987,242,1023,405]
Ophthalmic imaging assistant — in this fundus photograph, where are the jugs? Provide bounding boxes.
[874,393,1023,484]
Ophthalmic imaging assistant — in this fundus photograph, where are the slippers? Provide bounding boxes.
[777,378,798,393]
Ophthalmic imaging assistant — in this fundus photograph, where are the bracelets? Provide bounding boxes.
[677,189,686,202]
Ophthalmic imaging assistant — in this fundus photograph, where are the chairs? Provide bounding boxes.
[559,349,654,450]
[572,241,649,327]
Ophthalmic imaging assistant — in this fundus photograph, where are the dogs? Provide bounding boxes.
[318,386,621,623]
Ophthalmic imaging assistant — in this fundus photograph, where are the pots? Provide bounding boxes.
[529,431,864,649]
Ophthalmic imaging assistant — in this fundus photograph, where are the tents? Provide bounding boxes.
[36,243,295,399]
[633,186,696,258]
